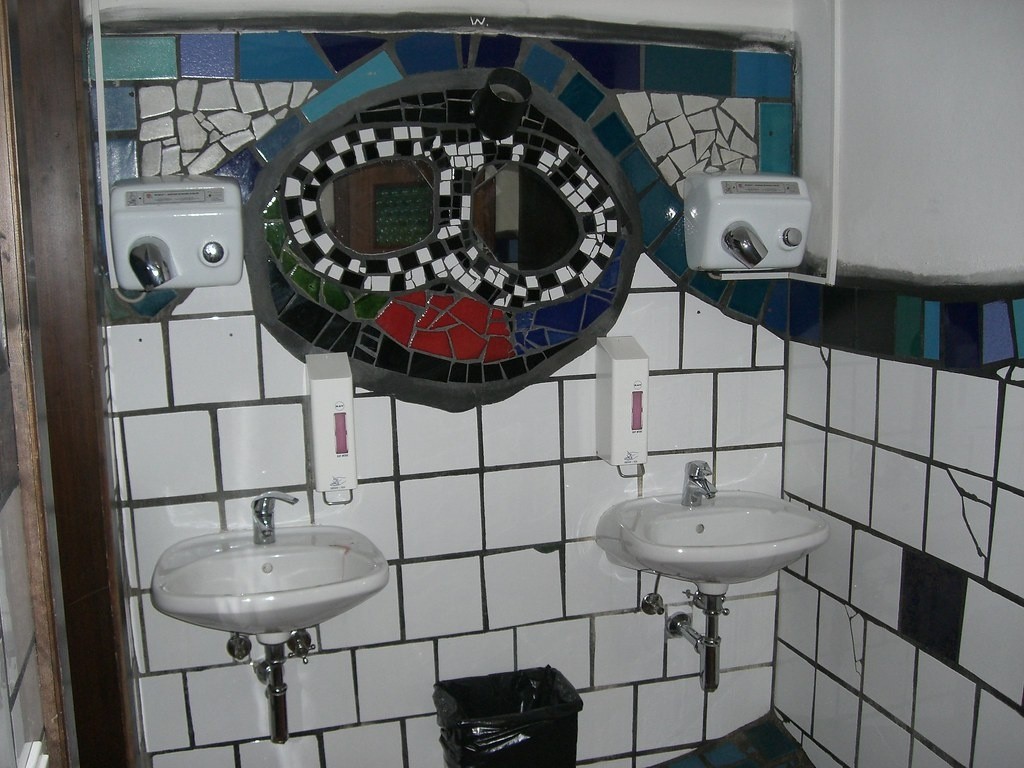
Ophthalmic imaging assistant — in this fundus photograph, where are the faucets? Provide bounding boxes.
[251,491,300,544]
[680,460,719,508]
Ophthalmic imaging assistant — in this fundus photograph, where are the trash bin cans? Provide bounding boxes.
[434,665,584,768]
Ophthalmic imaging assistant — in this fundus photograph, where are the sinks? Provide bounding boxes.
[151,524,388,647]
[594,492,830,596]
